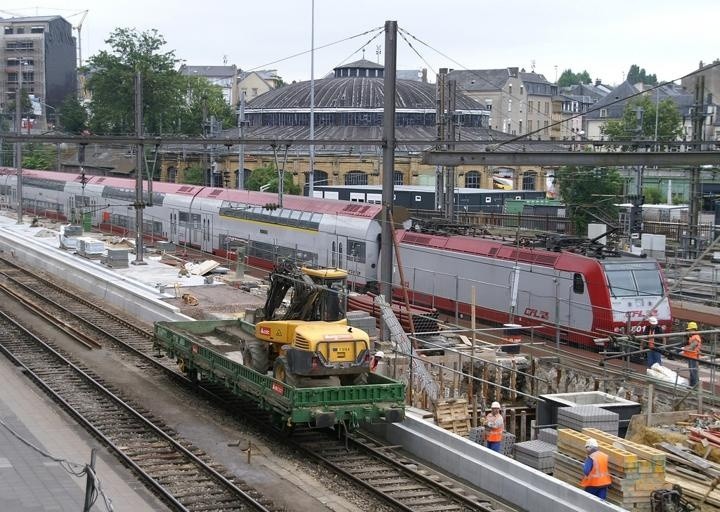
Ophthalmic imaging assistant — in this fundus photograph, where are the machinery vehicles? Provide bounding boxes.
[240,259,372,394]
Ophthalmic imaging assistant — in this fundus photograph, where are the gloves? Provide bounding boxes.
[639,349,643,354]
[479,418,489,425]
[678,347,685,354]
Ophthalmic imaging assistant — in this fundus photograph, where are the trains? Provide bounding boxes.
[0,167,674,361]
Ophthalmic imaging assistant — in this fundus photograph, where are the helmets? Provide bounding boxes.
[491,401,501,408]
[583,438,597,448]
[687,323,697,330]
[648,317,658,325]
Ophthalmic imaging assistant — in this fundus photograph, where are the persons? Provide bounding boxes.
[370,349,386,372]
[642,315,665,368]
[477,400,505,454]
[679,321,701,388]
[577,437,614,501]
